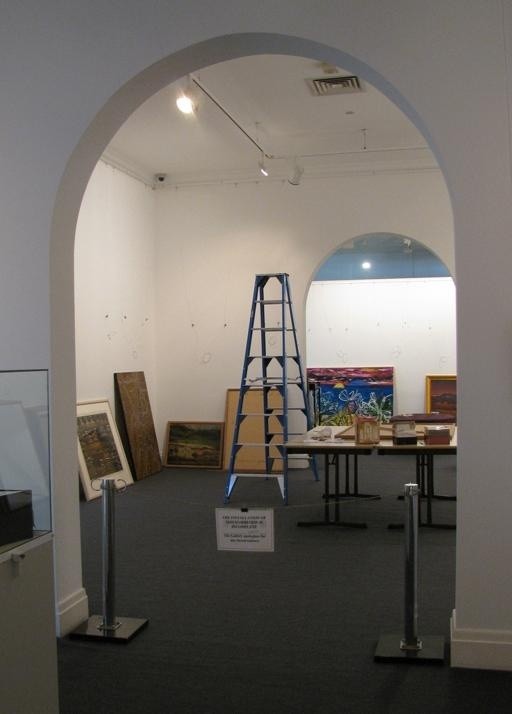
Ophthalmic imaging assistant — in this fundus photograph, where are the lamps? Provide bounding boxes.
[178,71,197,116]
[284,153,306,187]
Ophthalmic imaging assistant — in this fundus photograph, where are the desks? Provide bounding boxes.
[282,420,376,530]
[374,423,457,532]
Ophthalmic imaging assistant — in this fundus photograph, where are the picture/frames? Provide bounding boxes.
[76,399,133,503]
[424,375,457,416]
[164,419,226,469]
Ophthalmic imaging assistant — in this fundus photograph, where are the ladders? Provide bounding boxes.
[224,273,321,506]
